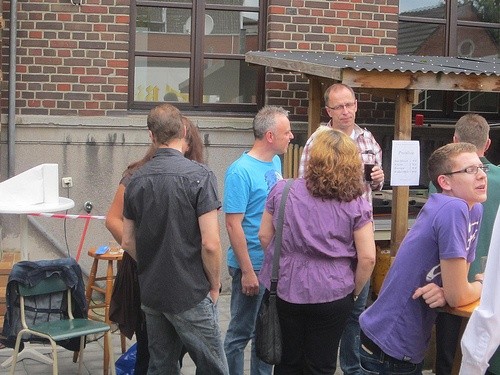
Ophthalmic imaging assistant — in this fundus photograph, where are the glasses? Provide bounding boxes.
[325,102,356,113]
[441,163,489,175]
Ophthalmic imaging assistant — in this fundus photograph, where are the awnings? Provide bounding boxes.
[179,60,254,97]
[243,48,500,94]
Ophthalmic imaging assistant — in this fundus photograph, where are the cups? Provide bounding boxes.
[364,161,375,181]
[480,257,487,273]
[108,241,118,254]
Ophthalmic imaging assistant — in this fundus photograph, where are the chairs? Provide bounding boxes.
[9,258,116,375]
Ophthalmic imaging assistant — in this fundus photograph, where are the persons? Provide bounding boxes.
[297,82,386,375]
[104,116,204,375]
[457,206,500,375]
[258,128,376,375]
[120,102,230,375]
[222,104,295,375]
[428,113,500,375]
[163,93,235,103]
[358,141,488,375]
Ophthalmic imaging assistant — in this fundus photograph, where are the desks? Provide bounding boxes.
[447,298,481,375]
[0,196,76,260]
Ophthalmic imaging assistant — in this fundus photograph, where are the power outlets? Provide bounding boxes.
[62,177,73,189]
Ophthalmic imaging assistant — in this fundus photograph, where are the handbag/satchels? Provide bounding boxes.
[255,291,281,365]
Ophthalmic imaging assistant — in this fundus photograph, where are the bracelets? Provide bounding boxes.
[474,279,483,286]
[352,294,359,301]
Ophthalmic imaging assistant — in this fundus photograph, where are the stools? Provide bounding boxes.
[73,248,127,375]
